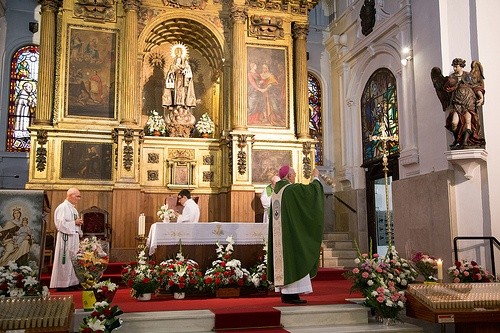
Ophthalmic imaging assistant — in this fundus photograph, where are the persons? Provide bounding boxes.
[161,41,196,108]
[260,165,325,304]
[431,58,486,150]
[50,187,83,288]
[175,189,200,222]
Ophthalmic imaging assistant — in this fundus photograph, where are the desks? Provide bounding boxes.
[147,222,268,269]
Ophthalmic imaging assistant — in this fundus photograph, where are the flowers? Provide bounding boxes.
[347,249,494,308]
[0,237,269,333]
[157,205,177,219]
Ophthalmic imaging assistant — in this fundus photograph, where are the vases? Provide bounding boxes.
[162,217,171,223]
[138,294,152,301]
[82,290,98,311]
[216,288,240,297]
[371,306,403,323]
[249,292,265,296]
[174,292,184,299]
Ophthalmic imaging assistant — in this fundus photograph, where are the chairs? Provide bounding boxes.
[78,206,112,245]
[44,231,55,266]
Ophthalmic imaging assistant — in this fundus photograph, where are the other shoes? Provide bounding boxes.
[282,297,307,304]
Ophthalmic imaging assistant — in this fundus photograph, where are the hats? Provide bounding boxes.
[278,165,289,179]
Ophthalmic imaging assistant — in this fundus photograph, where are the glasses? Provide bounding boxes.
[75,195,81,199]
[178,196,183,200]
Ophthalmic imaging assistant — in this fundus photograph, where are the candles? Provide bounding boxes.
[138,213,145,236]
